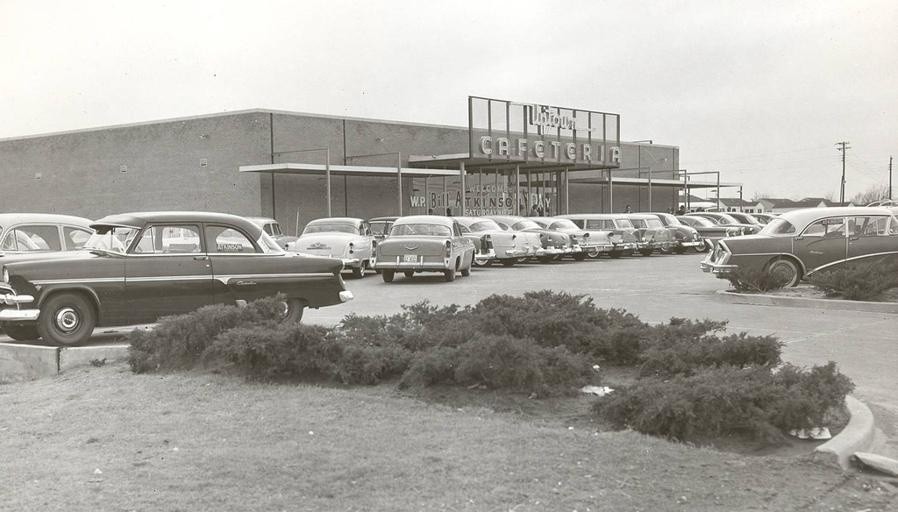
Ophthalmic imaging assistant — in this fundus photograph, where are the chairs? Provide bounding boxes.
[216,244,243,253]
[162,244,196,254]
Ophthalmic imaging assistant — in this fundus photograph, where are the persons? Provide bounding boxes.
[624,204,632,213]
[678,204,685,216]
[727,207,732,212]
[531,205,539,217]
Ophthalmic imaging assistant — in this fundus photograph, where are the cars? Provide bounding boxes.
[701,207,897,293]
[0,212,354,348]
[0,211,124,261]
